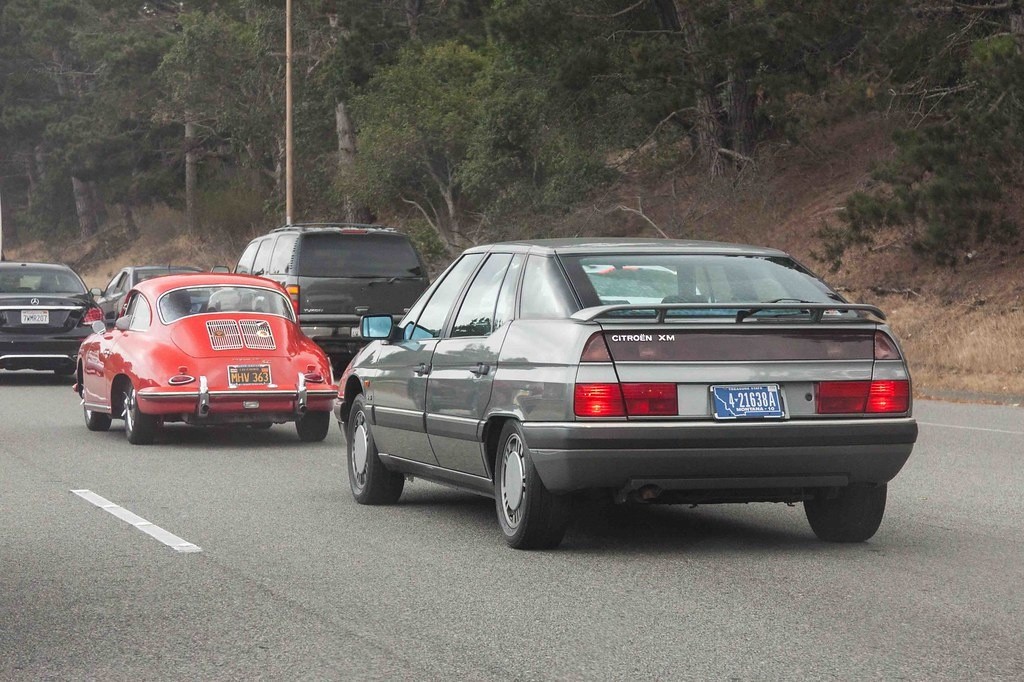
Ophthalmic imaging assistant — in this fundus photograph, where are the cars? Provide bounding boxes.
[90,263,207,329]
[72,273,341,446]
[1,261,100,376]
[332,237,919,550]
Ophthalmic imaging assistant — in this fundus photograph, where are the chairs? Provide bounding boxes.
[663,295,710,303]
[169,290,192,319]
[41,276,57,292]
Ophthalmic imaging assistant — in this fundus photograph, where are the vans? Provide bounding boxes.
[211,222,431,379]
